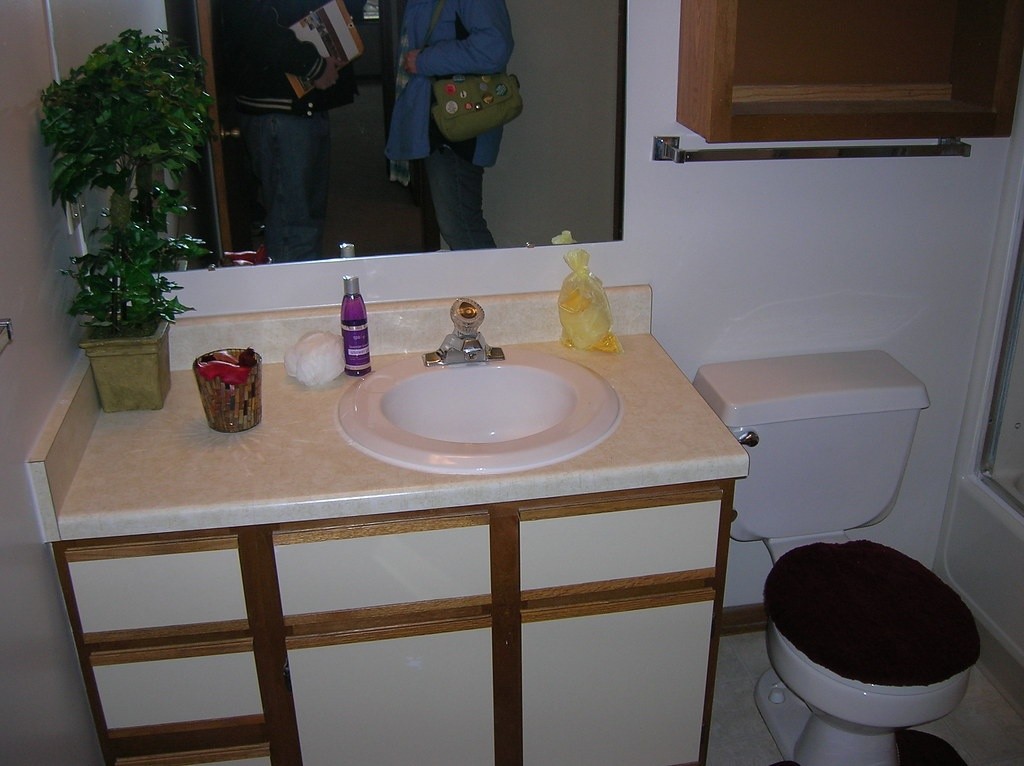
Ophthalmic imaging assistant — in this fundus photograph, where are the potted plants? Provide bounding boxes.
[34,29,173,415]
[158,233,206,273]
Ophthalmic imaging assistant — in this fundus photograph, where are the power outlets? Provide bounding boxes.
[77,192,89,221]
[65,194,81,234]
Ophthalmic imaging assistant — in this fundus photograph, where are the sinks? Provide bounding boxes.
[336,348,622,475]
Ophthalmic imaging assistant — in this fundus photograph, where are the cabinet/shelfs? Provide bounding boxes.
[675,0,1024,144]
[48,478,736,766]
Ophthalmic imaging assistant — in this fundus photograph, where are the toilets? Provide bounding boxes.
[690,347,982,765]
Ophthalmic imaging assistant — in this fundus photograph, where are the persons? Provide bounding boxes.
[220,0,366,262]
[383,0,514,251]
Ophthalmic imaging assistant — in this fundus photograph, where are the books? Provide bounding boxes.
[284,0,365,99]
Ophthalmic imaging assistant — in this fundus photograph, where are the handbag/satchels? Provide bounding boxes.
[423,0,524,143]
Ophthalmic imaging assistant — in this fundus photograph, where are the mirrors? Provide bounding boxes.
[43,0,628,286]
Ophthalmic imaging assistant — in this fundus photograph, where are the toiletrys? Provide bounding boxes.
[340,275,372,377]
[339,243,355,258]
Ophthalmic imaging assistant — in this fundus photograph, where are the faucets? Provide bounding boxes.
[421,297,505,367]
